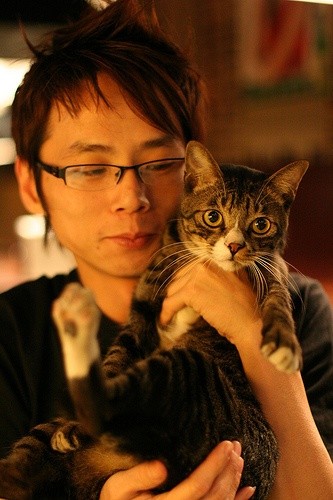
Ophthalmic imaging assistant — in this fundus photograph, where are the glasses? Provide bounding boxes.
[35,158,187,191]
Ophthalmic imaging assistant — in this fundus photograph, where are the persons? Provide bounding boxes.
[0,0,333,500]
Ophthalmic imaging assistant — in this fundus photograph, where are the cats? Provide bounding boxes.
[0,141,310,500]
